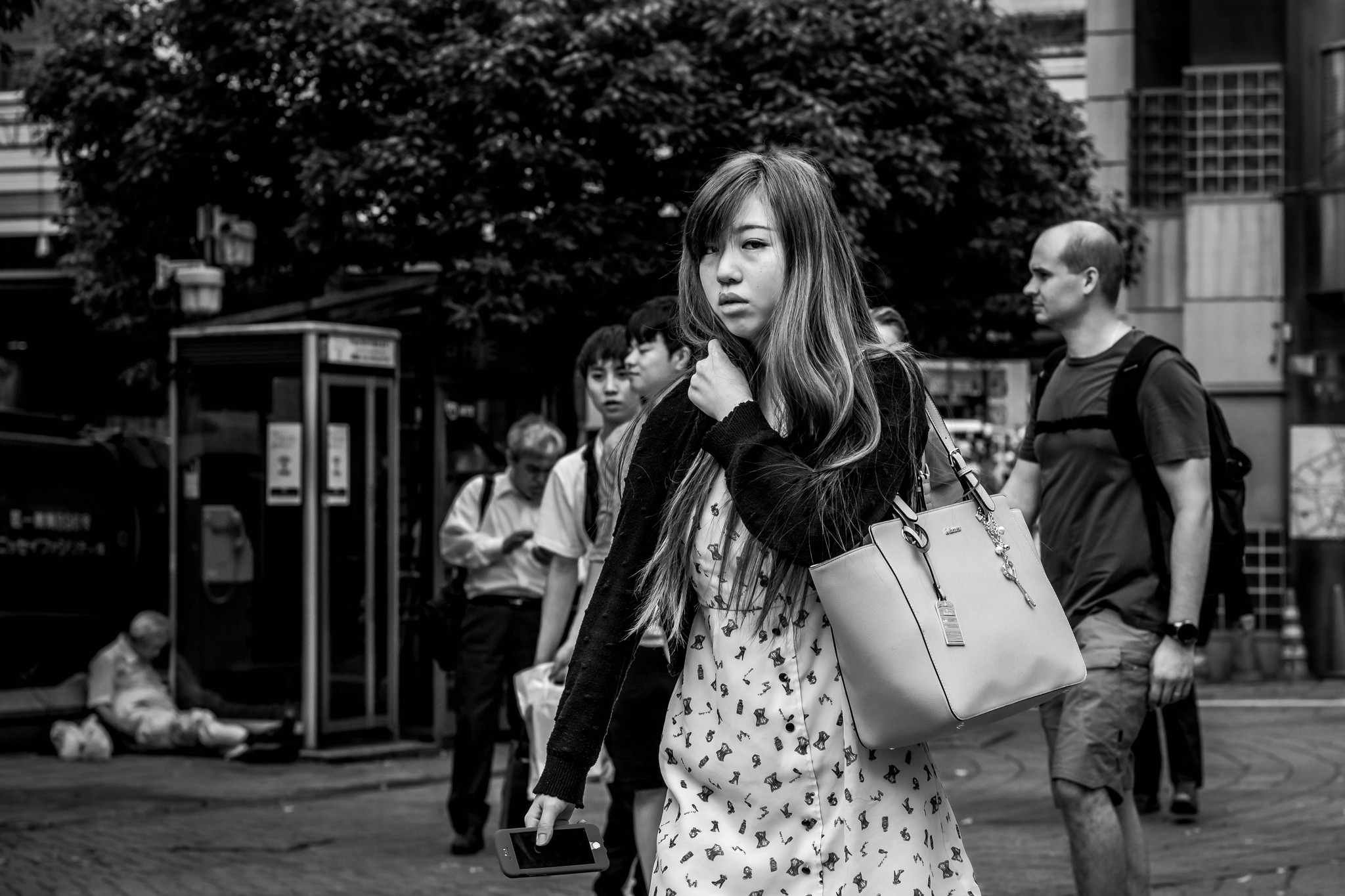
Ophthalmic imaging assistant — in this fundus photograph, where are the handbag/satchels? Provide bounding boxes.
[50,714,114,758]
[424,581,464,673]
[809,343,1087,749]
[512,663,603,800]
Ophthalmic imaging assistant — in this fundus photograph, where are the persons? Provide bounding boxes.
[526,321,646,892]
[974,220,1216,896]
[860,300,966,510]
[523,149,990,895]
[1130,675,1205,819]
[86,611,307,762]
[436,413,574,857]
[551,296,708,895]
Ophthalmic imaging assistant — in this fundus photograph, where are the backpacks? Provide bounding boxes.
[1035,346,1253,647]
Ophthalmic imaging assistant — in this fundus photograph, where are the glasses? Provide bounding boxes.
[526,464,552,474]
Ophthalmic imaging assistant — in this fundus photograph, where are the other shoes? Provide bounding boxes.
[1137,792,1162,816]
[274,710,296,743]
[282,722,304,763]
[1170,791,1199,815]
[451,831,485,853]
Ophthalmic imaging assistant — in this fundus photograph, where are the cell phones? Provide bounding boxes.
[493,824,610,878]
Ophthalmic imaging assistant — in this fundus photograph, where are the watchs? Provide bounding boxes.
[1163,620,1205,649]
